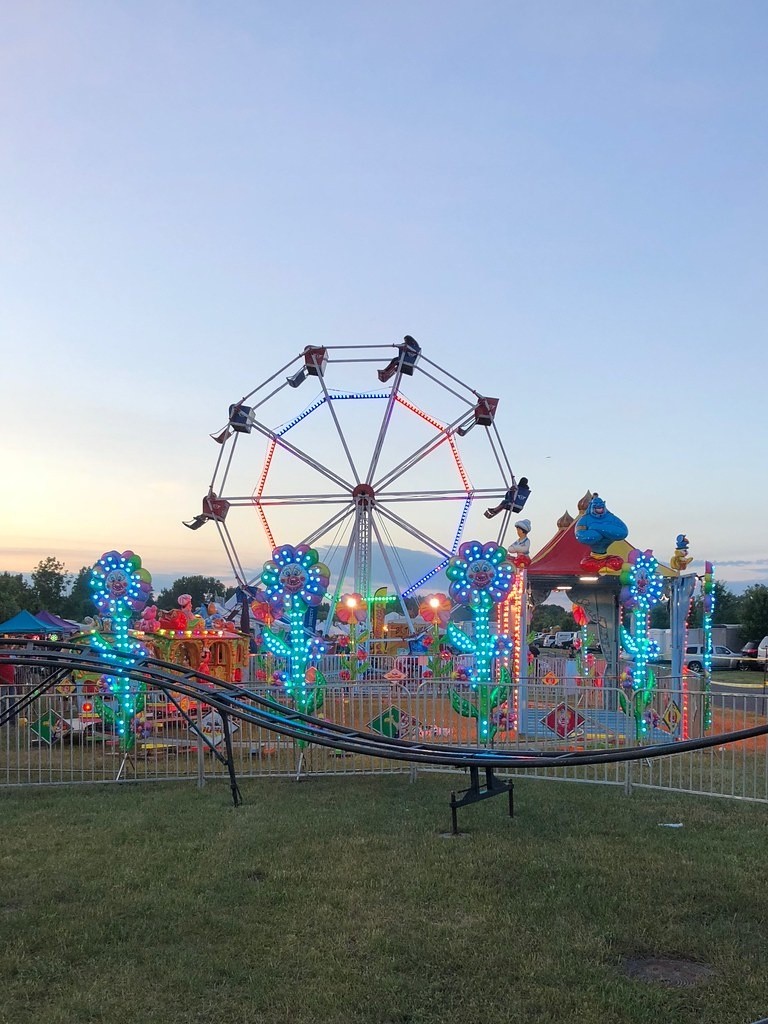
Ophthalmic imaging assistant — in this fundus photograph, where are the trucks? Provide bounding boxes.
[555,631,577,647]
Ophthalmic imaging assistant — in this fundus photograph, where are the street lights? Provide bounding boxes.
[419,593,454,677]
[336,593,369,679]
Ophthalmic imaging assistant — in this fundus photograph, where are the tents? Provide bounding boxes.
[0,609,91,679]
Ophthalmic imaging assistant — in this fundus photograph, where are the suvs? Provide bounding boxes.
[685,644,740,673]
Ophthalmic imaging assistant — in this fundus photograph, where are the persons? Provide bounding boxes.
[528,642,541,681]
[567,645,576,658]
[508,520,532,564]
[574,492,628,573]
[0,636,63,725]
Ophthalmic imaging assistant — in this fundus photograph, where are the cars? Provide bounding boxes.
[756,635,768,674]
[740,639,760,669]
[532,632,556,648]
